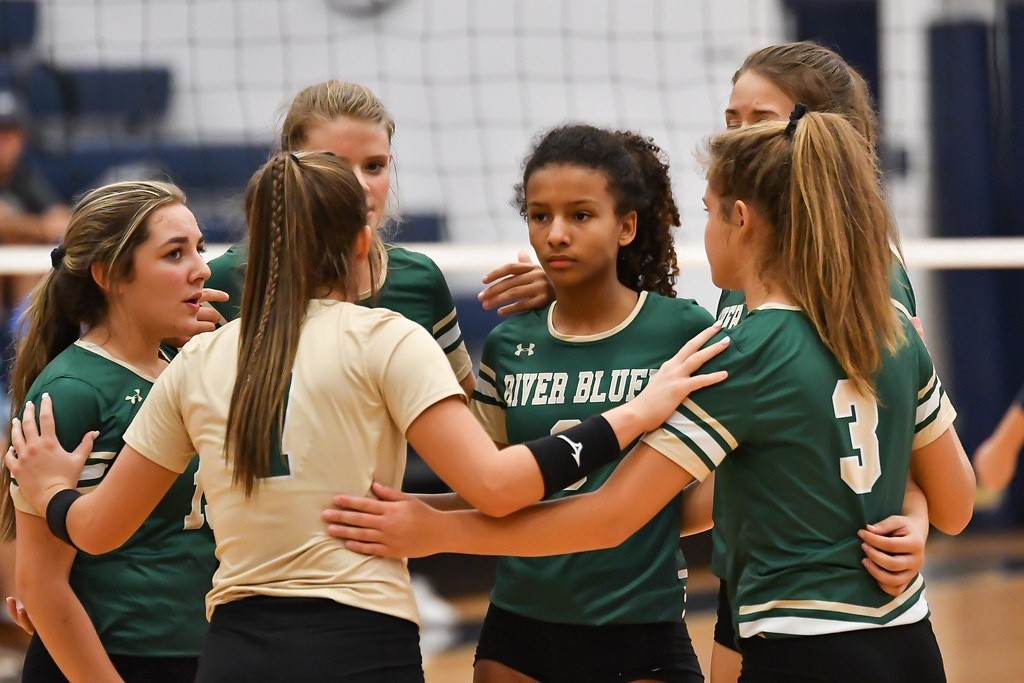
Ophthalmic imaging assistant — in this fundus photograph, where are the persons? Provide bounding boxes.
[320,101,978,683]
[0,43,1007,683]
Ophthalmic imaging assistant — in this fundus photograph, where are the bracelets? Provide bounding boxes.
[41,489,87,553]
